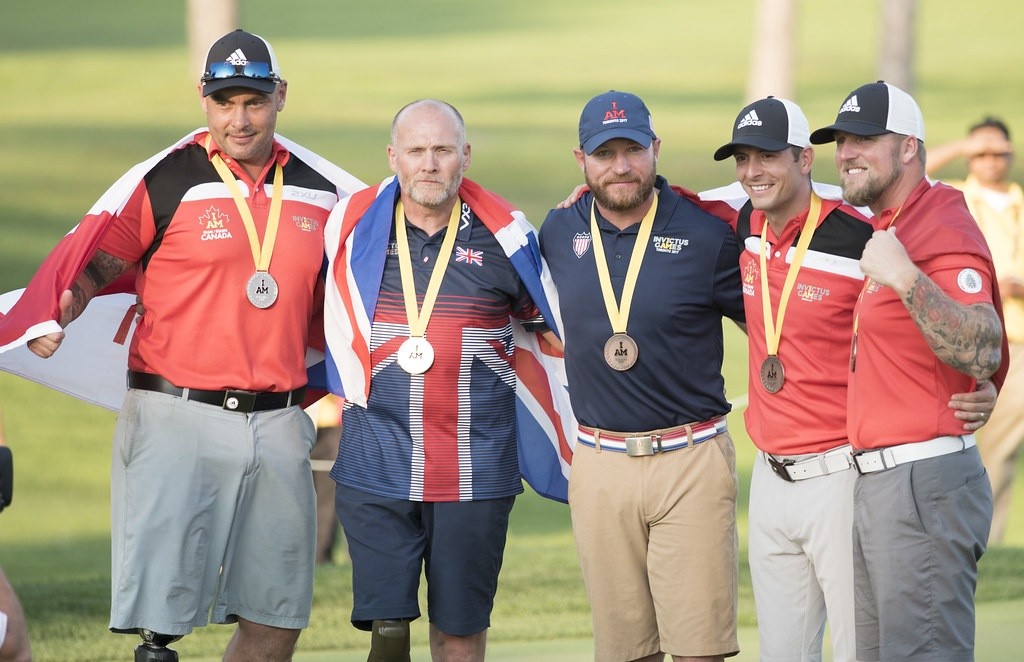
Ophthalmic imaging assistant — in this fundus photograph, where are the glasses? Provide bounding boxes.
[201,62,282,85]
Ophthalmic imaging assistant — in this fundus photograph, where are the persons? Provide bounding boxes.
[27,28,342,662]
[0,428,32,662]
[538,89,748,662]
[303,394,345,564]
[809,80,1004,662]
[925,117,1023,542]
[558,96,1010,662]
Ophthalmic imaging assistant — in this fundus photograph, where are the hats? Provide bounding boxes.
[200,28,281,98]
[809,79,924,146]
[714,95,811,162]
[578,90,656,156]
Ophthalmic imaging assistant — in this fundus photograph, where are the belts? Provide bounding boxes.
[758,442,853,483]
[849,433,978,475]
[578,414,729,456]
[128,369,306,413]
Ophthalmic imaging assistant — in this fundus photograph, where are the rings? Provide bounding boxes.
[980,412,985,421]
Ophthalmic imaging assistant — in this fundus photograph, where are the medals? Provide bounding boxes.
[759,356,786,394]
[604,333,638,371]
[850,335,858,371]
[398,337,434,374]
[247,272,279,309]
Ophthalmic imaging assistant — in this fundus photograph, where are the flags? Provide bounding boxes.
[0,126,580,504]
